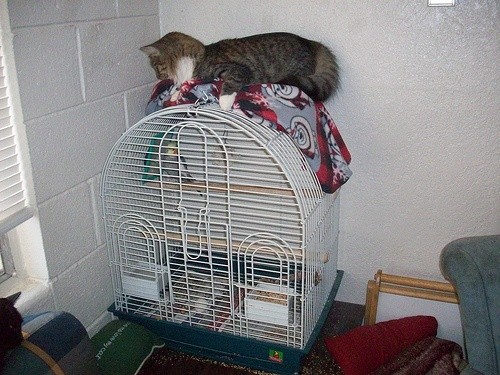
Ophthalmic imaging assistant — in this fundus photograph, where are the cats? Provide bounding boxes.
[138,31,344,112]
[0,291,23,364]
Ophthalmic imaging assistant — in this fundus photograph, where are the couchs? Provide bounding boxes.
[437,235,500,375]
